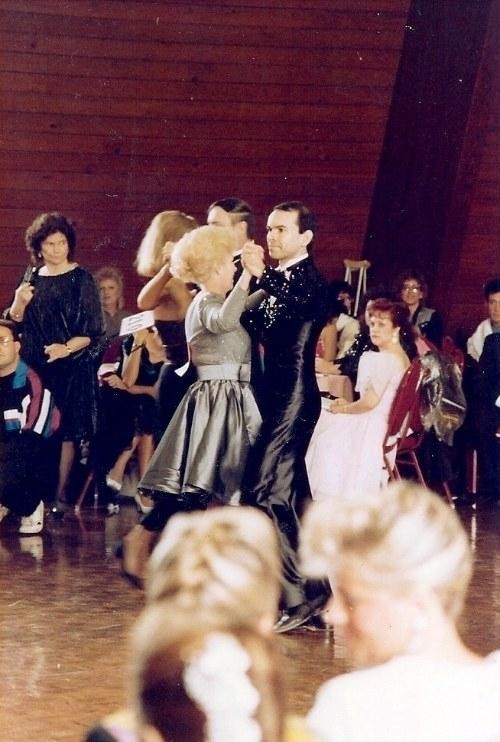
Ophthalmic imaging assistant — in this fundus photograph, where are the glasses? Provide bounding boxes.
[403,286,421,294]
[1,335,15,346]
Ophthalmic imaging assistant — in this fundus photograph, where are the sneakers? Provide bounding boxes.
[106,502,121,512]
[47,504,82,522]
[18,499,45,533]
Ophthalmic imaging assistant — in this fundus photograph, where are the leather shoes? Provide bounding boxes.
[273,600,330,635]
[105,469,123,491]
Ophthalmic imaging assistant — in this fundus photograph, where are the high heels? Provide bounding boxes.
[112,537,147,591]
[133,491,154,515]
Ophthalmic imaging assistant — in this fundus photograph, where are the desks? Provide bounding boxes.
[316,373,353,402]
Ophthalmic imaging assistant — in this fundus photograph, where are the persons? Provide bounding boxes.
[119,598,287,741]
[77,502,317,742]
[296,475,499,742]
[2,193,500,635]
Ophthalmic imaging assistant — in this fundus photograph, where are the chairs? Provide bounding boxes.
[381,356,443,487]
[441,337,463,509]
[73,335,121,511]
